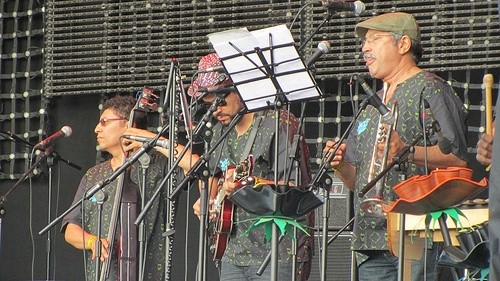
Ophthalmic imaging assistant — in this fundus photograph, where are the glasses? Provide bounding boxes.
[99,118,124,127]
[361,34,395,47]
[204,100,227,108]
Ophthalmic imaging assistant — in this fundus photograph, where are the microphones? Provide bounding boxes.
[198,66,227,74]
[306,41,331,68]
[34,126,72,149]
[424,99,452,155]
[356,74,395,124]
[123,135,170,149]
[197,86,237,92]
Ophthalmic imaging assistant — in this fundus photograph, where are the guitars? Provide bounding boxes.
[212,154,255,261]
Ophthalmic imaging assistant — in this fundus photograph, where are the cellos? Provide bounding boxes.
[96,87,158,281]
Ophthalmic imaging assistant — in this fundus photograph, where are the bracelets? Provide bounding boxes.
[255,176,261,185]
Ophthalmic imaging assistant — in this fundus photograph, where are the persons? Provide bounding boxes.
[61,96,200,281]
[324,13,469,281]
[476,87,500,166]
[188,53,314,281]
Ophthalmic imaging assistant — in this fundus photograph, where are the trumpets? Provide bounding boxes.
[361,81,399,220]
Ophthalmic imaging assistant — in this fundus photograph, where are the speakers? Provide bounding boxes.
[306,231,358,281]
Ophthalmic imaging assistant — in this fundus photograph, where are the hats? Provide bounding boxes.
[187,53,229,98]
[355,12,421,44]
